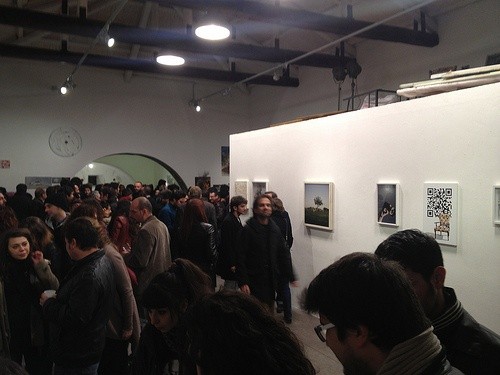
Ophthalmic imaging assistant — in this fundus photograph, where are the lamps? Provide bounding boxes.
[192,0,234,40]
[194,102,201,112]
[273,70,281,81]
[100,34,115,48]
[156,49,186,66]
[61,79,77,95]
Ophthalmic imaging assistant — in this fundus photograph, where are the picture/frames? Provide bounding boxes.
[302,181,334,231]
[234,180,249,210]
[376,181,400,227]
[251,180,269,209]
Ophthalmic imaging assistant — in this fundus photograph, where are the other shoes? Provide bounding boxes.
[284,315,291,322]
[277,304,284,312]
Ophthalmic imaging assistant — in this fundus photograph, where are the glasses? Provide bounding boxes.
[313,323,336,342]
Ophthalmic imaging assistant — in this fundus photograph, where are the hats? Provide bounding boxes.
[45,192,68,212]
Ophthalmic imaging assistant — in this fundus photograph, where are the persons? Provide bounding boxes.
[375,228,500,375]
[296,252,464,375]
[0,177,315,375]
